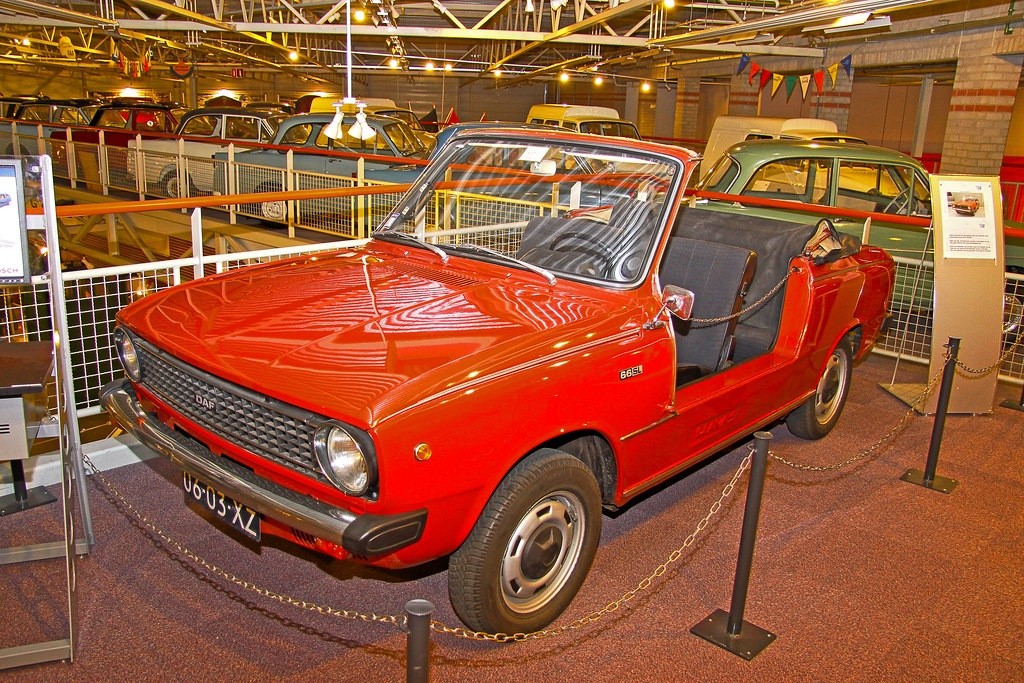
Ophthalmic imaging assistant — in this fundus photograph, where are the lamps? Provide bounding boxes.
[323,0,376,140]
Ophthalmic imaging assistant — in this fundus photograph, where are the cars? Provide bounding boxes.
[0,88,674,246]
[672,138,1023,341]
[953,195,979,216]
[699,114,932,217]
[96,121,900,644]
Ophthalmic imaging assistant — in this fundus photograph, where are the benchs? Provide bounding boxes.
[516,217,758,370]
[609,196,861,366]
